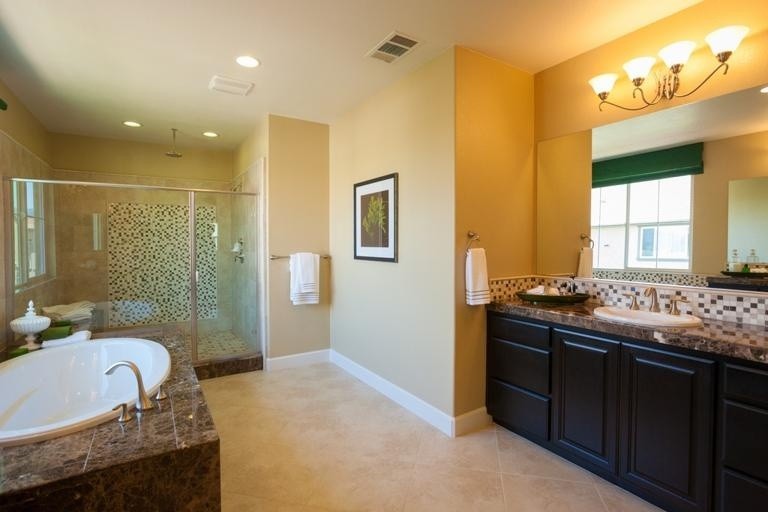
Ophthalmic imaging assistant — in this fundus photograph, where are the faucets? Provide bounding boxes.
[643,287,660,313]
[105,361,153,411]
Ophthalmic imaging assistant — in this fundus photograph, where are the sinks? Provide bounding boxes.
[593,306,705,328]
[0,337,171,440]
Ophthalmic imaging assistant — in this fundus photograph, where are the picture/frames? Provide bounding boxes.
[354,172,398,262]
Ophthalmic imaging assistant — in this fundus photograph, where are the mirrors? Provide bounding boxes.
[535,83,768,294]
[727,177,768,265]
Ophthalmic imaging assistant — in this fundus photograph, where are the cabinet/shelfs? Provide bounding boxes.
[485,311,768,511]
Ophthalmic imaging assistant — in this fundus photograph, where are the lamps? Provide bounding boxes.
[587,24,749,111]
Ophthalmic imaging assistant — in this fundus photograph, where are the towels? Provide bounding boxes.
[289,252,321,306]
[576,246,593,278]
[465,247,491,305]
